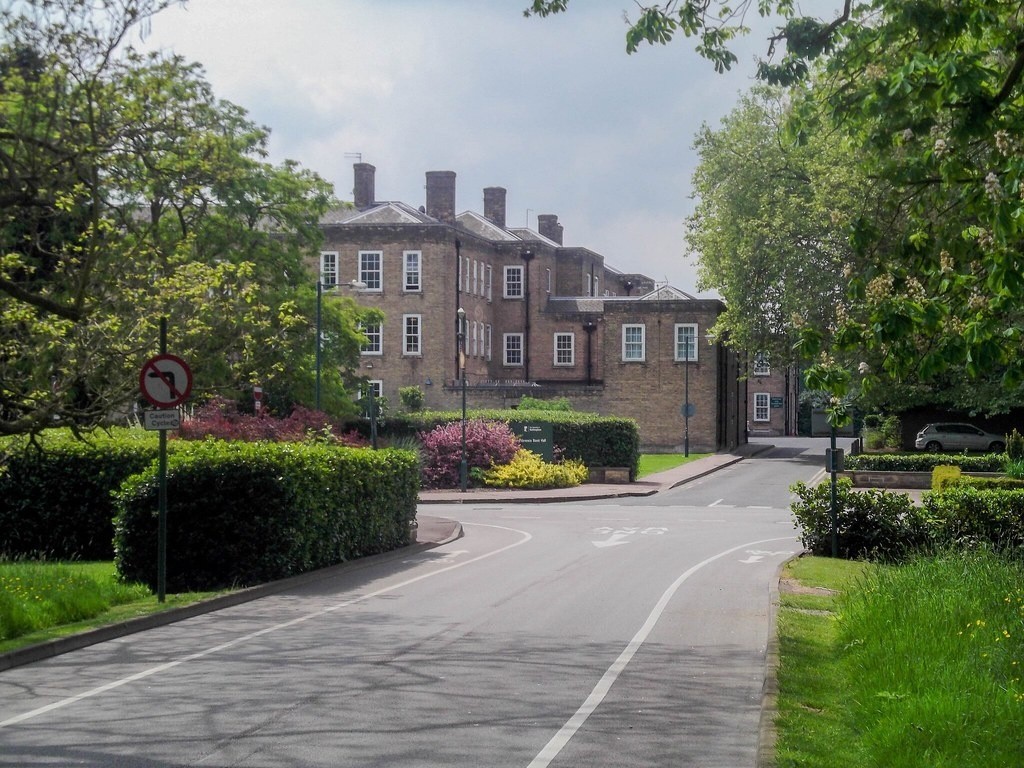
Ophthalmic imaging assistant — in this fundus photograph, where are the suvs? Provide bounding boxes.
[915,422,1007,453]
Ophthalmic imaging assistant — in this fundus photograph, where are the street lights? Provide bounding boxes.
[457,307,466,492]
[316,279,368,411]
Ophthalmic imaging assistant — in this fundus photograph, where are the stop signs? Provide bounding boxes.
[253,383,263,401]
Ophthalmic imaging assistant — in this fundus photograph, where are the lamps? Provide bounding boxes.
[425,376,432,387]
[365,361,372,368]
